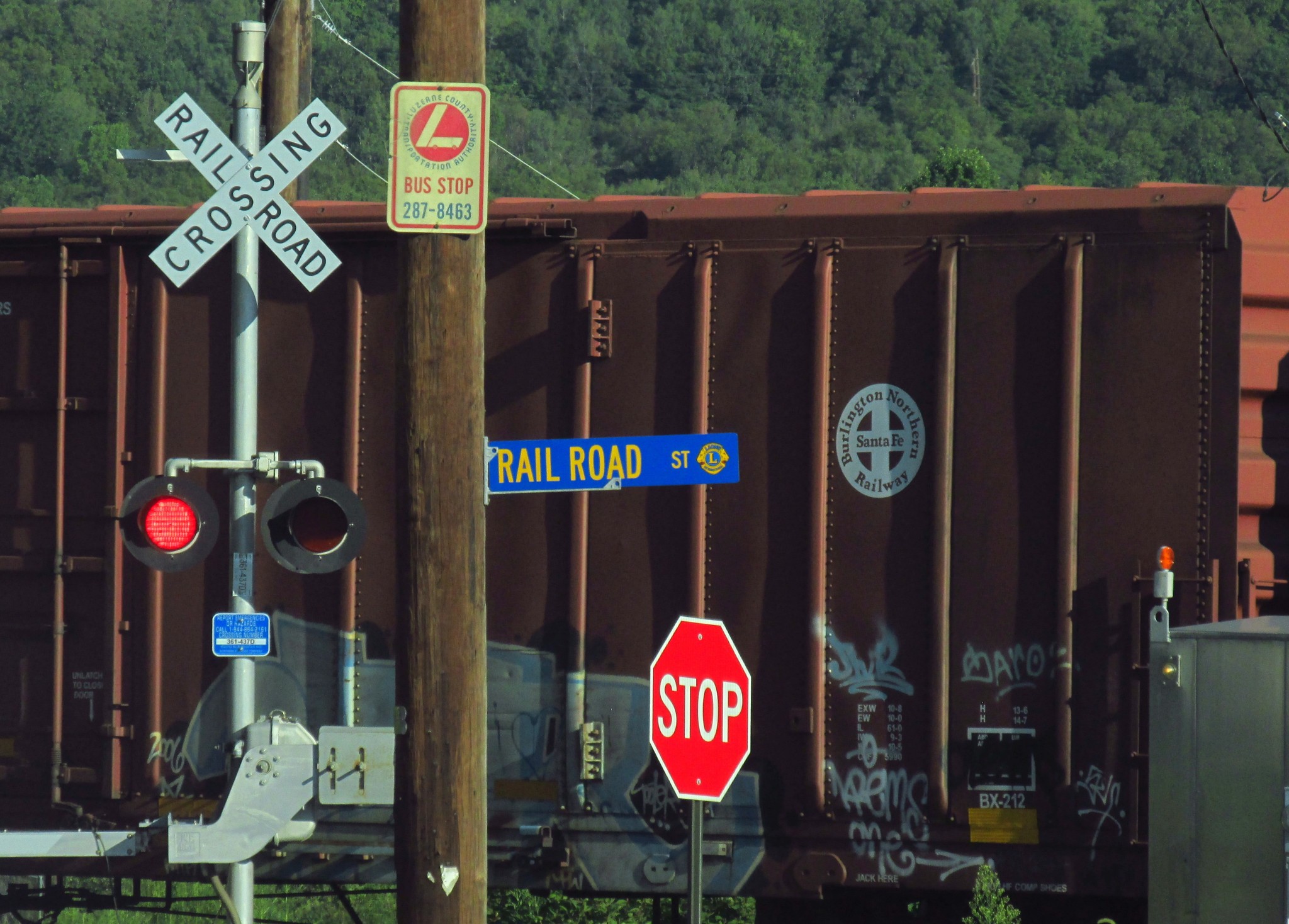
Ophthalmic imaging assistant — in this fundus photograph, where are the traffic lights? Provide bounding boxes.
[266,476,365,574]
[123,474,220,574]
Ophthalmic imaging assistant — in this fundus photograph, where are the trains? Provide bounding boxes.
[0,181,1289,924]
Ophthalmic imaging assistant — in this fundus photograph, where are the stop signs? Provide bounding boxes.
[650,615,751,802]
